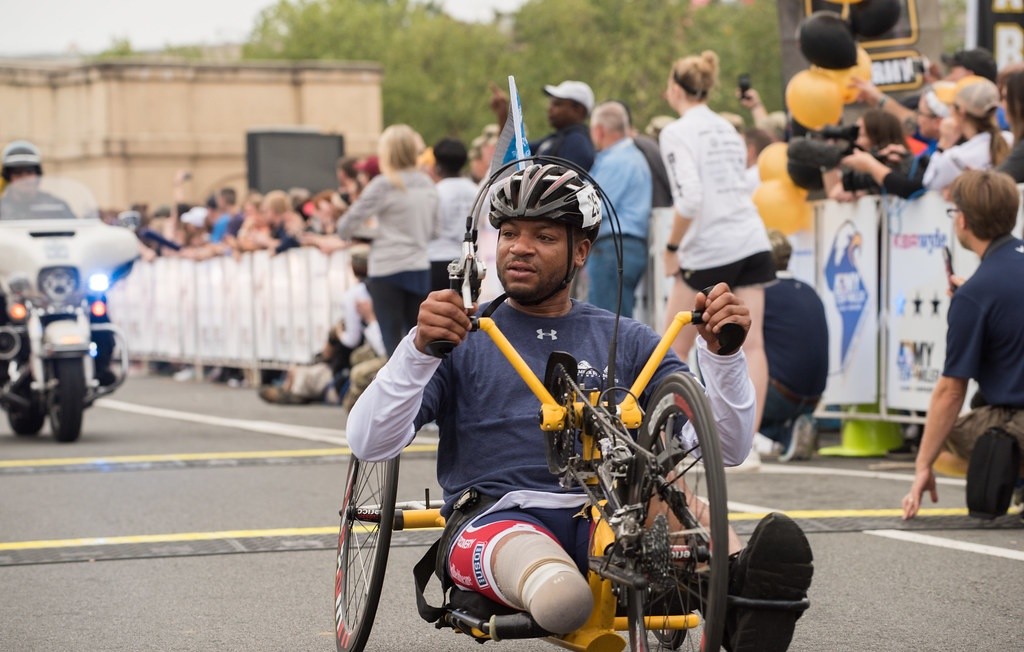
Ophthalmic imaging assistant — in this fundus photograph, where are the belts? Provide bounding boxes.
[769,378,818,405]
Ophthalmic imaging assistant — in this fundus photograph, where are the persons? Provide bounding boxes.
[0,140,115,388]
[87,169,273,388]
[335,124,441,359]
[899,168,1024,521]
[756,228,829,461]
[429,138,480,291]
[344,162,815,651]
[658,50,777,468]
[720,45,1024,453]
[470,76,672,319]
[252,147,443,407]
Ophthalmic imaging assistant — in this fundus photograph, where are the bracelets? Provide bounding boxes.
[877,96,889,108]
[666,244,678,252]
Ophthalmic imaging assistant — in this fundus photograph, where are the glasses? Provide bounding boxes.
[946,208,962,218]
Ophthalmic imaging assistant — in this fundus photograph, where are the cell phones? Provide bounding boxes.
[738,73,752,100]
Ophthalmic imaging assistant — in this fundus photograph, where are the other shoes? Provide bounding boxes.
[94,370,115,386]
[720,511,814,652]
[778,414,816,461]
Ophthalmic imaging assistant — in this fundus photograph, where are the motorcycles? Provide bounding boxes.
[0,176,144,442]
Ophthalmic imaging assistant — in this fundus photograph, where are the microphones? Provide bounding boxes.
[788,138,844,168]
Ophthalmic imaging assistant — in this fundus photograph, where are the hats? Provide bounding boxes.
[939,48,996,83]
[543,80,595,115]
[931,76,1000,120]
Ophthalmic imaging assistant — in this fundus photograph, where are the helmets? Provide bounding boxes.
[2,142,42,181]
[488,164,602,230]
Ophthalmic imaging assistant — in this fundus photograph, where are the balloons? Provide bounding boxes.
[799,15,860,69]
[753,180,810,233]
[809,45,872,103]
[850,0,901,39]
[756,141,810,201]
[784,70,842,131]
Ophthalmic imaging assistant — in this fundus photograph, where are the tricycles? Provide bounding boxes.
[333,288,747,652]
